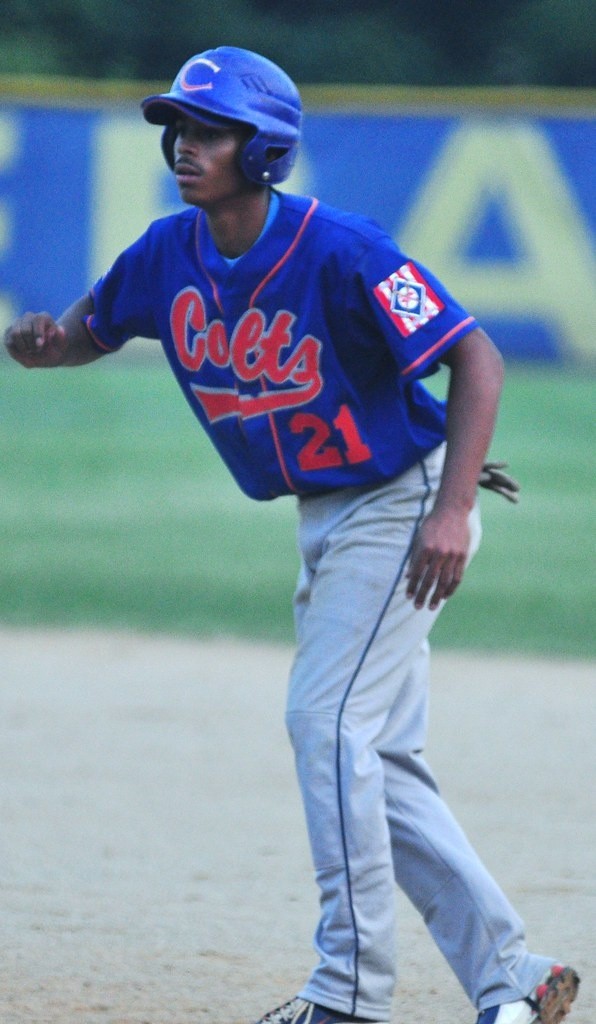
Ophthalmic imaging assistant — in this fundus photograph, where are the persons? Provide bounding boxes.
[3,47,582,1024]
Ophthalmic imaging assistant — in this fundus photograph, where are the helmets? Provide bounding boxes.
[140,46,303,186]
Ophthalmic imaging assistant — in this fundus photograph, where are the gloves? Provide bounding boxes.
[479,460,517,500]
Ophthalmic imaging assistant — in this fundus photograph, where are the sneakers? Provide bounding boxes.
[475,965,583,1024]
[255,995,377,1024]
